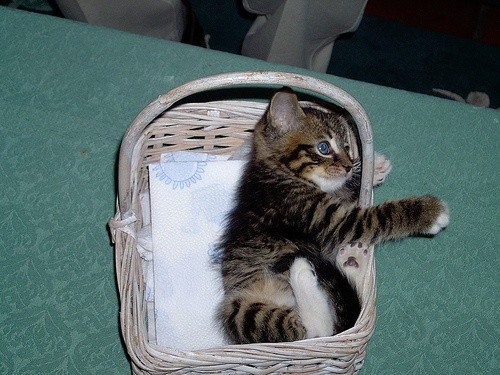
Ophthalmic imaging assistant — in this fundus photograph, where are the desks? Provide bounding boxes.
[0,6,500,375]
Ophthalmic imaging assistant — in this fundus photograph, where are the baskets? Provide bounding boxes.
[107,70,375,375]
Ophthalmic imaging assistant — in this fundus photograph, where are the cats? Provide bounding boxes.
[213,86,451,346]
